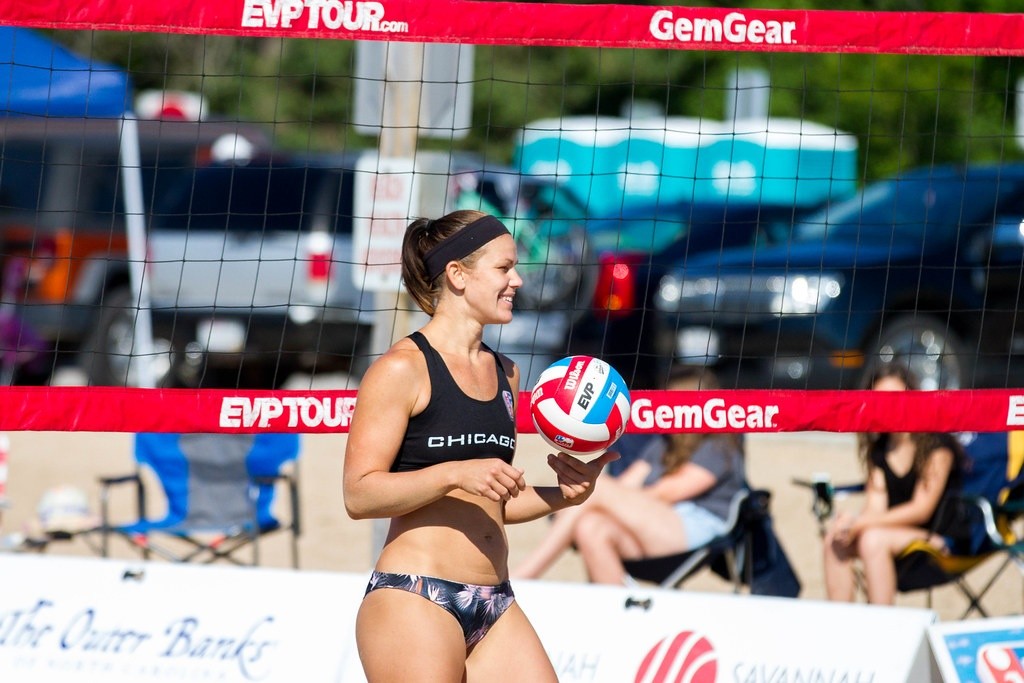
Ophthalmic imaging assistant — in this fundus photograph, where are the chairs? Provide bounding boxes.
[813,426,1024,620]
[96,431,302,565]
[607,431,772,595]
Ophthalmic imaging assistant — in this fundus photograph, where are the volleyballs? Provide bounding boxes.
[529,354,633,456]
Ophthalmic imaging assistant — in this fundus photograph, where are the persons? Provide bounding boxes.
[823,364,973,605]
[343,210,623,683]
[517,366,745,588]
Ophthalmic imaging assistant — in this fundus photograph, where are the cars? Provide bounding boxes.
[1,114,1024,389]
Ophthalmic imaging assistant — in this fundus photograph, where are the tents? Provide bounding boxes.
[0,25,156,388]
